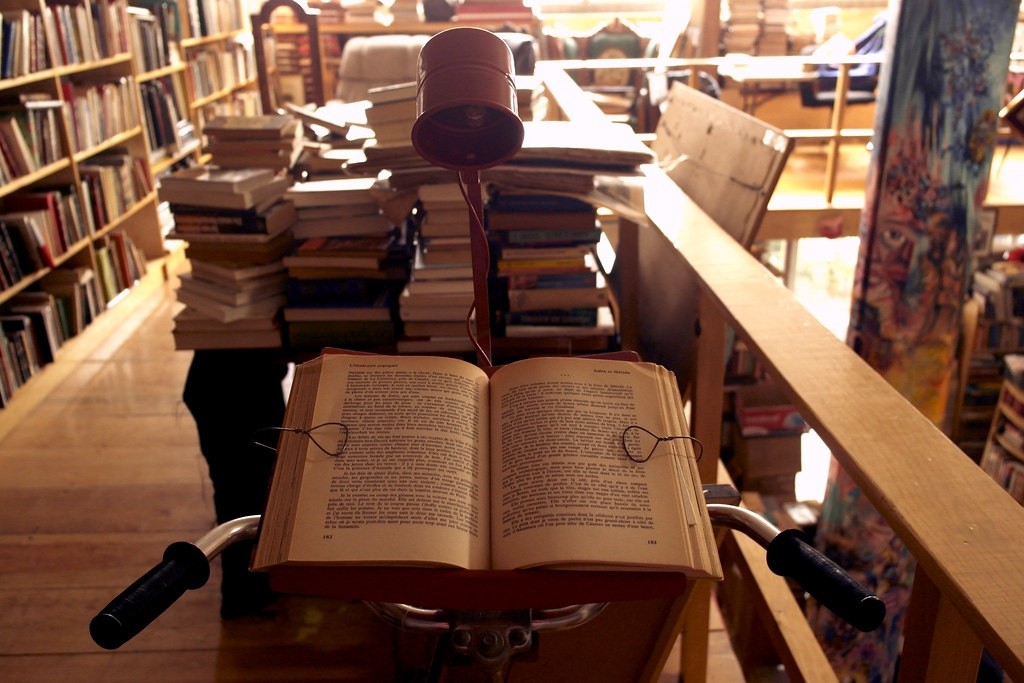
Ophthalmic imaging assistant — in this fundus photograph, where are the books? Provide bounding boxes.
[0,0,661,415]
[711,3,824,92]
[927,231,1023,507]
[248,351,727,585]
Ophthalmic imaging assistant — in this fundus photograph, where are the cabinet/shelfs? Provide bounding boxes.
[0,0,929,419]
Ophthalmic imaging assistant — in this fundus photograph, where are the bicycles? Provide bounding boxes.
[89,294,887,682]
